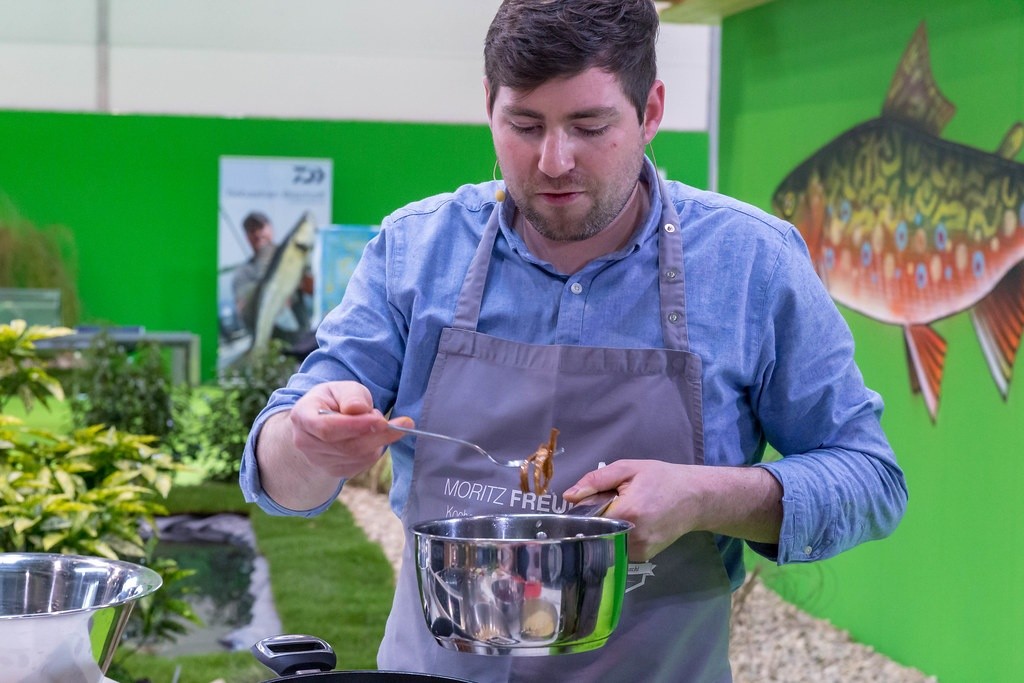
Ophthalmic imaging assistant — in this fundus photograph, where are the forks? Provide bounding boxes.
[319,409,565,468]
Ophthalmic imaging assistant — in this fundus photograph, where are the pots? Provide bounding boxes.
[408,489,636,658]
[250,632,476,683]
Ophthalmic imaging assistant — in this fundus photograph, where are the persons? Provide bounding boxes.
[233,212,313,357]
[237,0,909,683]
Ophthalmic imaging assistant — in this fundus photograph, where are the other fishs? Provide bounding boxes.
[245,212,315,367]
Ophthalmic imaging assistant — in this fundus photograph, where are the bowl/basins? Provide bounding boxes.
[0,551,164,683]
